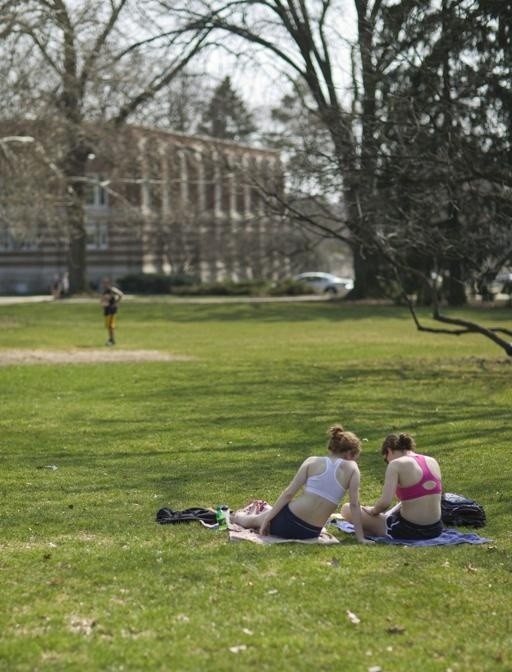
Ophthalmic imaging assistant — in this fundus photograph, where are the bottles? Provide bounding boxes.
[215,506,231,530]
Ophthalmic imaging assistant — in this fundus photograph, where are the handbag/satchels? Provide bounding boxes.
[440,490,485,526]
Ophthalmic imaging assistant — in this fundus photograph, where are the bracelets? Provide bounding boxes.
[370,507,379,515]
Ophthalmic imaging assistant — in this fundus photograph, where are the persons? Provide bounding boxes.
[49,271,67,301]
[340,433,444,540]
[100,278,125,346]
[206,422,376,544]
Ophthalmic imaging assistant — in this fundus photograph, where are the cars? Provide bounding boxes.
[293,272,354,294]
